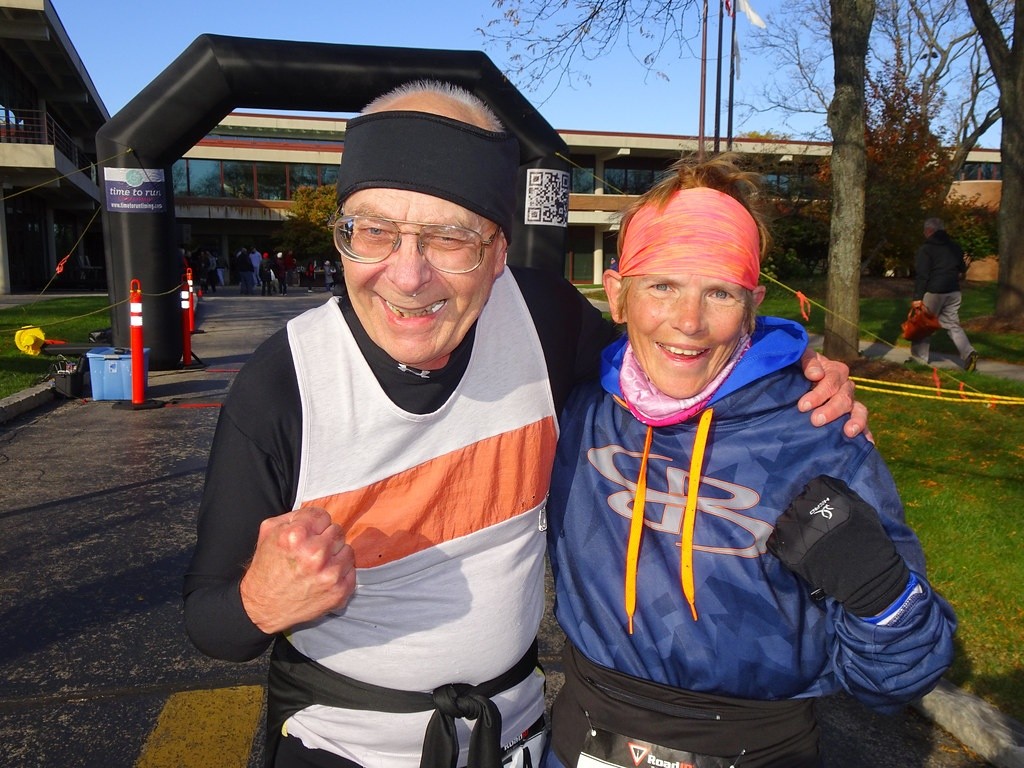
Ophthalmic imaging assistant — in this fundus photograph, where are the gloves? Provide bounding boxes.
[763,475,914,620]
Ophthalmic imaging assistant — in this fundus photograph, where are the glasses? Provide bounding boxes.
[326,201,503,274]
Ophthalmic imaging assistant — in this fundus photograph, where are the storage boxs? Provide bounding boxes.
[53,373,84,398]
[86,345,152,400]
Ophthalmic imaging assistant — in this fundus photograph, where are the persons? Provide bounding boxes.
[608,257,619,275]
[724,0,767,30]
[912,218,979,375]
[181,82,879,768]
[537,154,957,768]
[180,246,348,297]
